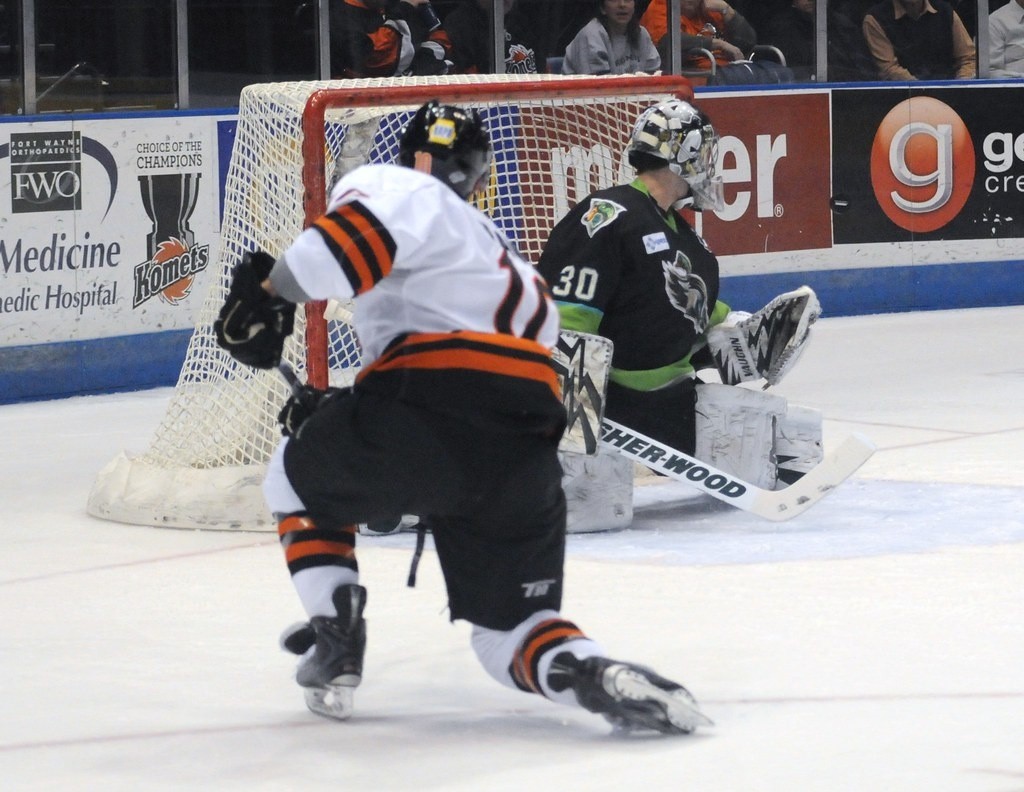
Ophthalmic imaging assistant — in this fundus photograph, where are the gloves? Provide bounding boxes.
[211,251,297,369]
[714,286,821,383]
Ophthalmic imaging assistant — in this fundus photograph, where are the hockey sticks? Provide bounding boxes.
[240,310,312,404]
[599,416,880,525]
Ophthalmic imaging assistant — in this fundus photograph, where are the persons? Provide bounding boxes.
[295,0,453,79]
[560,0,662,75]
[759,0,860,66]
[639,0,757,67]
[213,97,711,735]
[862,0,976,81]
[974,0,1024,79]
[534,98,876,523]
[443,1,552,74]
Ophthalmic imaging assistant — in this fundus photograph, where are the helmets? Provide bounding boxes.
[399,99,492,199]
[628,98,724,211]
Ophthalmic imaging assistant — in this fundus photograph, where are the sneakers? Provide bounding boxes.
[283,582,367,718]
[547,652,717,735]
